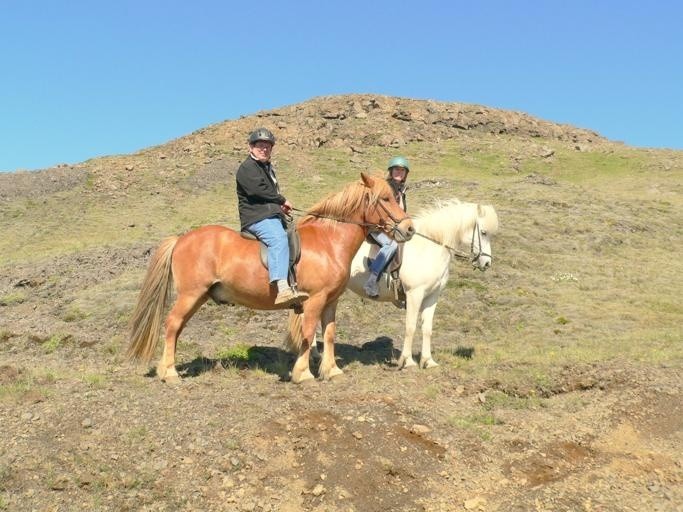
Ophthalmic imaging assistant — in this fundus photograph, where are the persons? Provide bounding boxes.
[237,128,309,309]
[363,156,410,299]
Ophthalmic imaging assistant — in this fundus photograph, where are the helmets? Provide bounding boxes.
[248,128,275,145]
[388,156,409,172]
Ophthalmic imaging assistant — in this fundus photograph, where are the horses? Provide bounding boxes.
[124,172,415,384]
[310,196,498,369]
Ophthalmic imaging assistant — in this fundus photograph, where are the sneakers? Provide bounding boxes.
[274,289,309,305]
[364,280,379,297]
